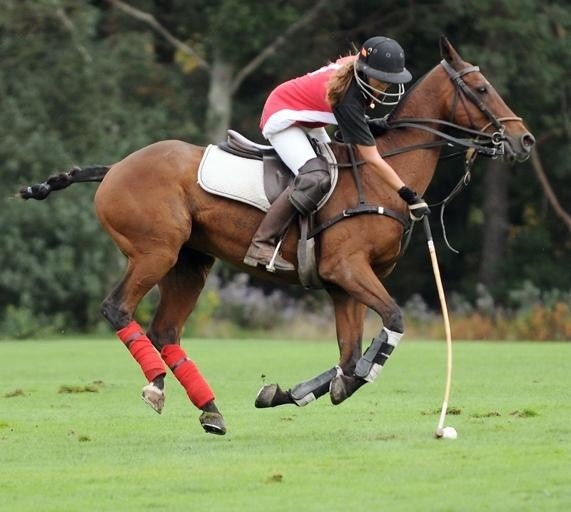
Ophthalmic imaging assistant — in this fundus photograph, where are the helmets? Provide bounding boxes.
[353,35,413,107]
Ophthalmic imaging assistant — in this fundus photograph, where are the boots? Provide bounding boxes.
[241,185,298,272]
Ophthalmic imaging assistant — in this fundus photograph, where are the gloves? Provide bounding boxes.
[397,185,432,218]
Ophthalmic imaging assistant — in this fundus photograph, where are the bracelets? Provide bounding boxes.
[399,188,414,201]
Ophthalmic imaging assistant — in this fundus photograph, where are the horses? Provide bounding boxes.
[17,30,536,435]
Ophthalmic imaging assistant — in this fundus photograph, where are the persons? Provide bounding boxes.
[243,36,431,272]
